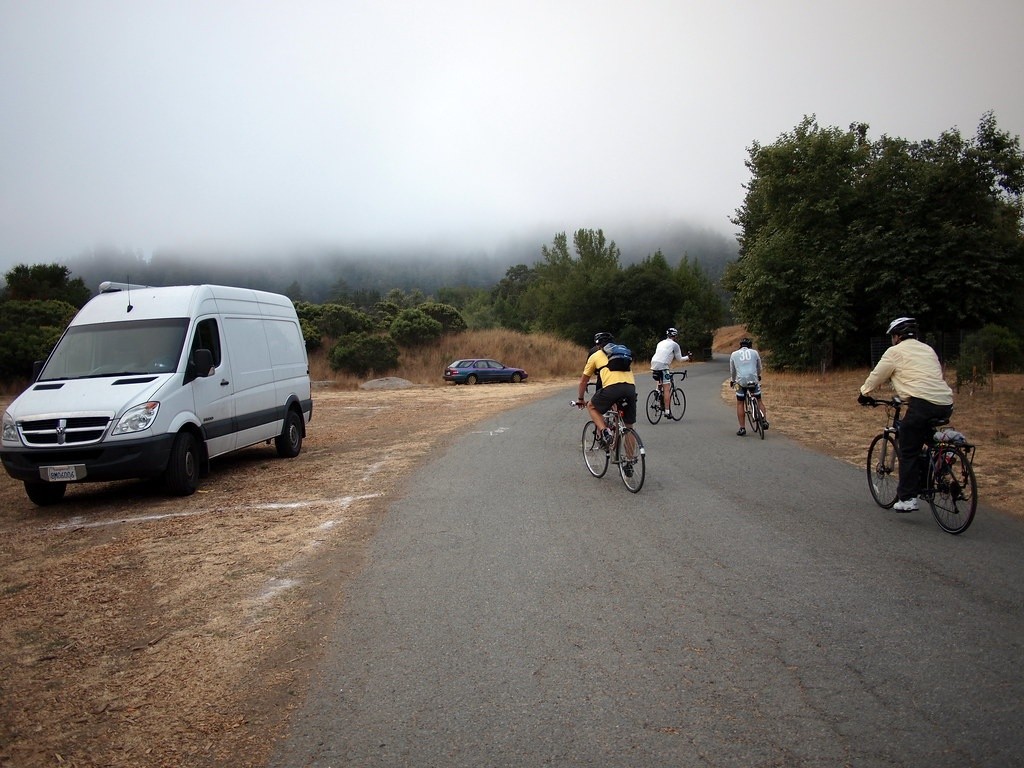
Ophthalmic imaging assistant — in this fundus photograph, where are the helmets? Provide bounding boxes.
[886,317,918,334]
[665,328,678,336]
[598,333,616,345]
[740,338,752,347]
[594,333,600,344]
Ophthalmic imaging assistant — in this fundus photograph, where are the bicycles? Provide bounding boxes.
[570,400,647,494]
[645,370,688,425]
[731,377,769,440]
[860,394,978,536]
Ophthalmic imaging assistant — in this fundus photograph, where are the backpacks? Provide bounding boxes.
[602,342,633,371]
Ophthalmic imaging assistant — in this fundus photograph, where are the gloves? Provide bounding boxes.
[686,353,693,359]
[758,376,761,380]
[730,381,733,387]
[856,393,870,405]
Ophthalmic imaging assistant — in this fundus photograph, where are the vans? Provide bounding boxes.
[0,281,314,509]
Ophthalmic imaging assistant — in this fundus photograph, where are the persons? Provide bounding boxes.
[577,332,637,477]
[730,338,769,435]
[857,318,954,511]
[651,328,690,417]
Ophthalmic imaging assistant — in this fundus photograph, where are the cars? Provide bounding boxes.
[443,358,528,385]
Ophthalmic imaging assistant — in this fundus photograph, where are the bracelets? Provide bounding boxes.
[578,398,584,400]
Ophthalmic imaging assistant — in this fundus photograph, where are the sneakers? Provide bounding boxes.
[763,422,769,430]
[941,451,956,472]
[737,429,746,435]
[893,498,919,511]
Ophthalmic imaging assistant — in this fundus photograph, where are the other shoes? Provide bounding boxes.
[599,431,612,448]
[664,411,672,418]
[655,390,660,400]
[624,465,634,477]
